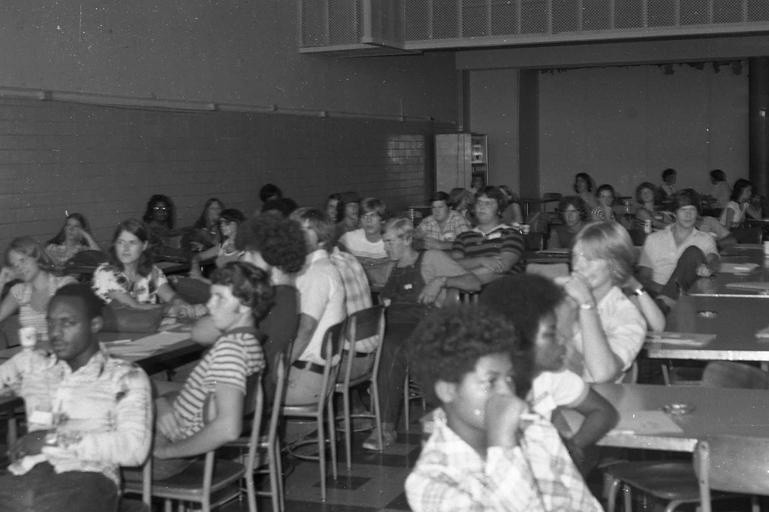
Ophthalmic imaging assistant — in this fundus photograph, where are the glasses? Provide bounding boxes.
[360,214,382,219]
[475,200,498,207]
[152,206,168,211]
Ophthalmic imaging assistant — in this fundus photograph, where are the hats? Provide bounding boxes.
[341,193,361,203]
[671,188,699,210]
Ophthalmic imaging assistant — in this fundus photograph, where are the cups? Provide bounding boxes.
[19,327,36,351]
[521,224,530,236]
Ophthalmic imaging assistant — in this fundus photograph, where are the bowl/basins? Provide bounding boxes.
[661,403,695,415]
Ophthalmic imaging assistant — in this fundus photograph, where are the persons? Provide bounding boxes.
[416,192,472,251]
[553,221,647,385]
[452,185,529,284]
[447,188,474,218]
[337,198,397,304]
[121,262,276,482]
[477,272,621,490]
[703,170,733,217]
[635,182,673,245]
[43,213,104,272]
[588,184,618,224]
[695,215,738,252]
[658,169,680,210]
[0,237,79,348]
[188,219,308,438]
[142,195,213,278]
[633,189,721,313]
[194,197,227,244]
[572,172,598,208]
[340,190,361,235]
[326,194,342,221]
[282,207,348,407]
[191,209,248,283]
[91,219,206,319]
[0,284,154,511]
[721,178,762,243]
[261,199,301,218]
[496,186,524,226]
[546,196,601,251]
[259,184,280,202]
[327,241,375,384]
[363,216,481,450]
[616,275,667,334]
[405,303,606,512]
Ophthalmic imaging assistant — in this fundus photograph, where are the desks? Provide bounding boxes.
[521,252,570,263]
[0,277,221,447]
[686,272,769,298]
[155,256,217,274]
[646,294,769,387]
[566,382,768,495]
[714,244,769,274]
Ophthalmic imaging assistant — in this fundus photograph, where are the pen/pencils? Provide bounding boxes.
[606,430,635,437]
[103,338,135,346]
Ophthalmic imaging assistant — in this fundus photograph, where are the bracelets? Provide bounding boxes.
[580,302,595,310]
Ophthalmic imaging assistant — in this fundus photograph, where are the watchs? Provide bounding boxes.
[438,276,450,286]
[633,287,647,298]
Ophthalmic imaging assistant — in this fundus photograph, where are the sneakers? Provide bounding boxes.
[363,426,397,450]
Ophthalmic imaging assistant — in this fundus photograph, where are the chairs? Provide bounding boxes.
[370,289,460,430]
[63,251,116,282]
[122,368,264,512]
[300,303,386,471]
[179,333,293,512]
[118,454,152,512]
[611,196,634,220]
[542,193,563,225]
[601,362,769,512]
[240,317,348,502]
[693,434,768,512]
[526,199,545,217]
